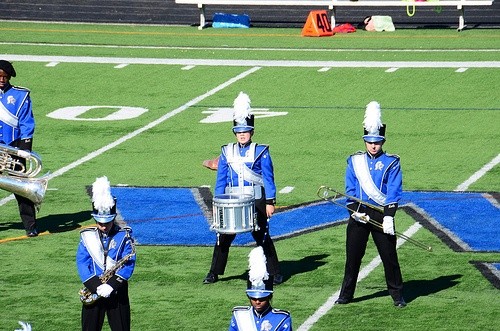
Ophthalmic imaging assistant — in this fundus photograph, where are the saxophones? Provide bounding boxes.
[79,238,136,306]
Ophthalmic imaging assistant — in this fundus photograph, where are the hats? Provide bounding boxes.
[247,246,274,301]
[91,176,118,223]
[362,100,386,145]
[0,60,16,77]
[232,92,254,133]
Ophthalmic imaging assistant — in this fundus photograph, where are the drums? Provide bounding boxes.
[211,193,256,234]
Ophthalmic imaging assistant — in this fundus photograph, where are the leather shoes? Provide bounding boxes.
[26,228,37,236]
[335,297,350,304]
[393,296,406,307]
[272,273,283,284]
[203,273,218,283]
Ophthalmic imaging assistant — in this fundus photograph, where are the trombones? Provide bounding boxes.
[316,185,433,252]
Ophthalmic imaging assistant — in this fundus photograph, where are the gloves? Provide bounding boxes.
[382,216,395,235]
[95,283,114,297]
[350,212,370,223]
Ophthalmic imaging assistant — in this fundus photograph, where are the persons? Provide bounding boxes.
[0,60,38,237]
[203,91,284,285]
[229,245,292,331]
[335,101,407,308]
[75,175,136,331]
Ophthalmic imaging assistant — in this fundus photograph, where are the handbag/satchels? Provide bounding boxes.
[357,15,371,30]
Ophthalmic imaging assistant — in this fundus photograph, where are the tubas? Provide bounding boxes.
[0,142,50,214]
